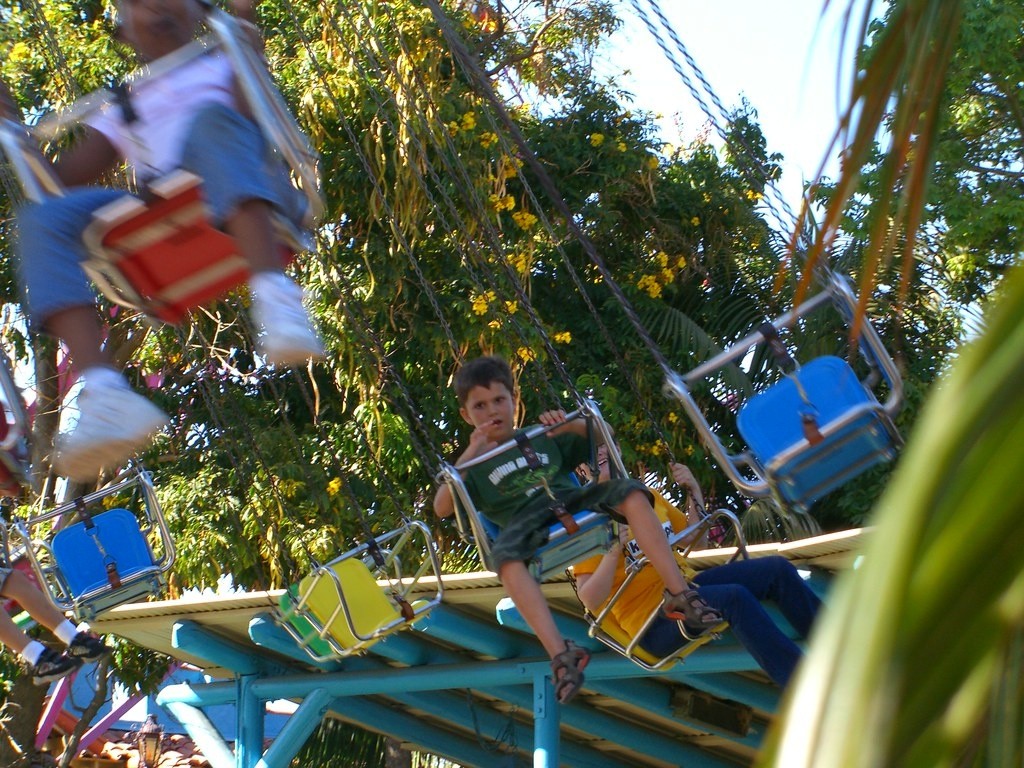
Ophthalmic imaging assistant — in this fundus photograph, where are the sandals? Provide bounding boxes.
[662,587,724,630]
[550,639,591,706]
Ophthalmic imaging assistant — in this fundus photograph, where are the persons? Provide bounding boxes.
[1,0,324,687]
[571,443,824,693]
[432,356,731,708]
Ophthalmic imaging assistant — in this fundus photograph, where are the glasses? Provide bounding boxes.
[600,443,607,448]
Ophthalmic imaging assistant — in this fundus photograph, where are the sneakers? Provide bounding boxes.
[52,382,171,481]
[32,647,83,686]
[66,632,112,663]
[246,271,325,368]
